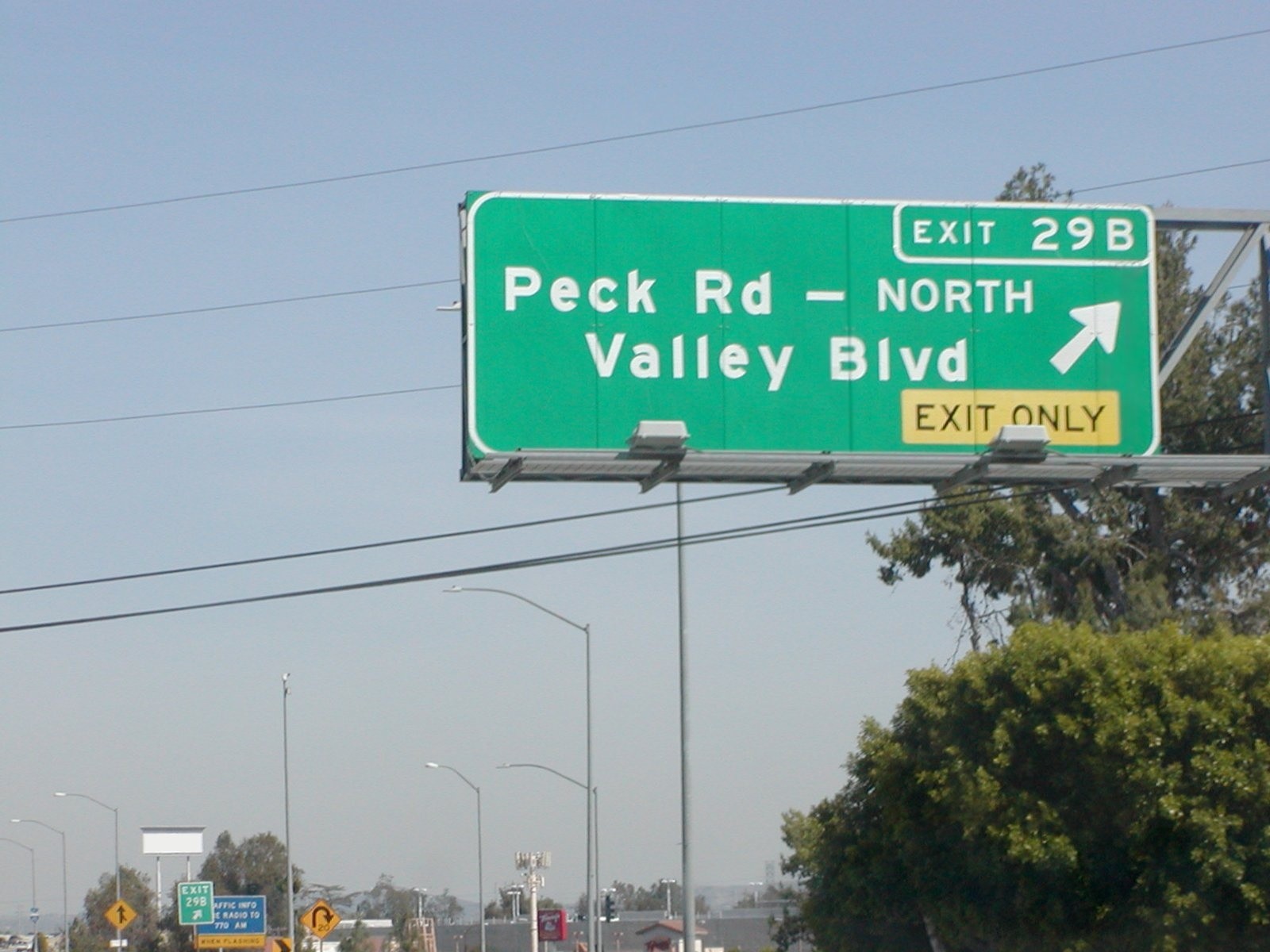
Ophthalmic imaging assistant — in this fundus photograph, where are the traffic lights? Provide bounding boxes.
[605,897,615,924]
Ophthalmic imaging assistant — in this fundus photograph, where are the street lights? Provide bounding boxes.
[281,672,292,952]
[55,793,122,952]
[496,763,604,952]
[413,888,427,919]
[464,923,488,952]
[514,849,551,952]
[10,819,69,952]
[444,586,594,952]
[507,884,524,922]
[661,879,676,920]
[425,763,486,952]
[749,882,764,909]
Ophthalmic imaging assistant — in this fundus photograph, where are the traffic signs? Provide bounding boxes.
[177,881,216,925]
[302,900,341,939]
[460,191,1162,463]
